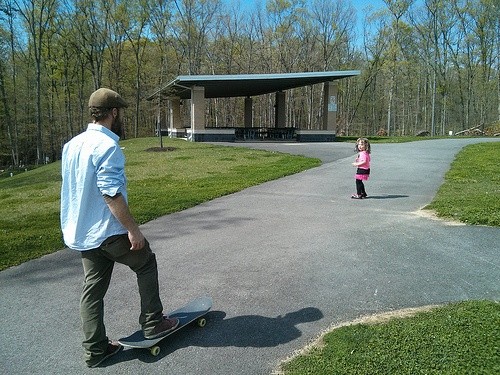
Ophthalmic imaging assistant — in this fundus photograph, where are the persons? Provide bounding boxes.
[352,138,371,199]
[60,88,179,368]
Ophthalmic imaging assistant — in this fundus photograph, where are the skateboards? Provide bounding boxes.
[118,298,213,356]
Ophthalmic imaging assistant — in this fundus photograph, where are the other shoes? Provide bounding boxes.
[143,316,179,339]
[86,339,121,367]
[351,194,361,198]
[361,192,367,197]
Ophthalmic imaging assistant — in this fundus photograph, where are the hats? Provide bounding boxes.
[88,87,129,108]
